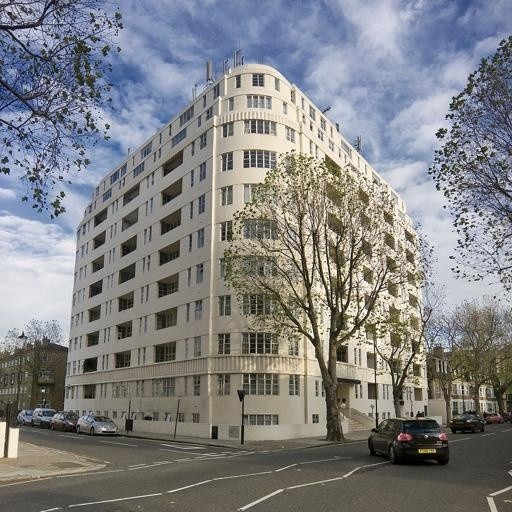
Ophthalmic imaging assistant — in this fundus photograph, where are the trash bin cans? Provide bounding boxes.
[125,419,133,434]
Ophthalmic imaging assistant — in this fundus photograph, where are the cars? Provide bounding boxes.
[17,408,118,436]
[450,414,485,433]
[484,412,512,425]
[368,417,449,465]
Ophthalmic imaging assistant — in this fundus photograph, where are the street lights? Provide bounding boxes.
[18,331,27,366]
[41,386,46,408]
